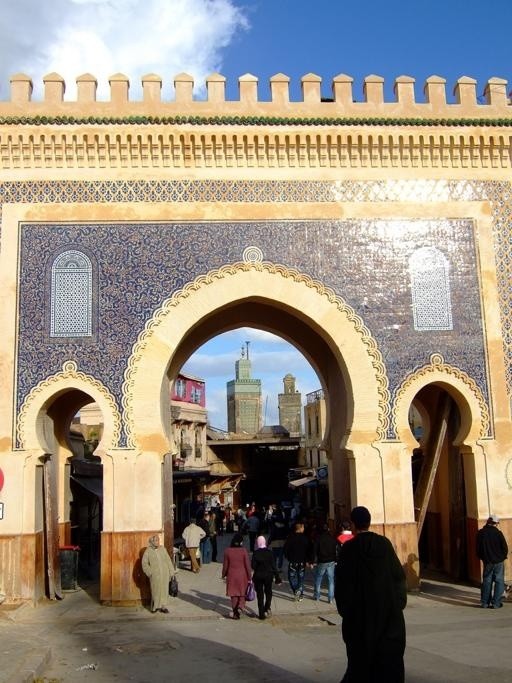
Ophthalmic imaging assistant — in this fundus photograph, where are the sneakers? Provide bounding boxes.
[314,598,333,603]
[483,603,503,609]
[295,591,303,602]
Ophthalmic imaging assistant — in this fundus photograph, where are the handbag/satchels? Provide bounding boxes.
[246,577,255,601]
[169,575,178,597]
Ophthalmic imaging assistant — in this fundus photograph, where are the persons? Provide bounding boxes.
[142,535,174,613]
[475,515,508,608]
[334,506,407,683]
[181,500,337,620]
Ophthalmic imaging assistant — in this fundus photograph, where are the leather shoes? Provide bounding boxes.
[152,608,168,613]
[260,608,271,620]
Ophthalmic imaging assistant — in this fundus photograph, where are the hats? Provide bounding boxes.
[488,516,499,523]
